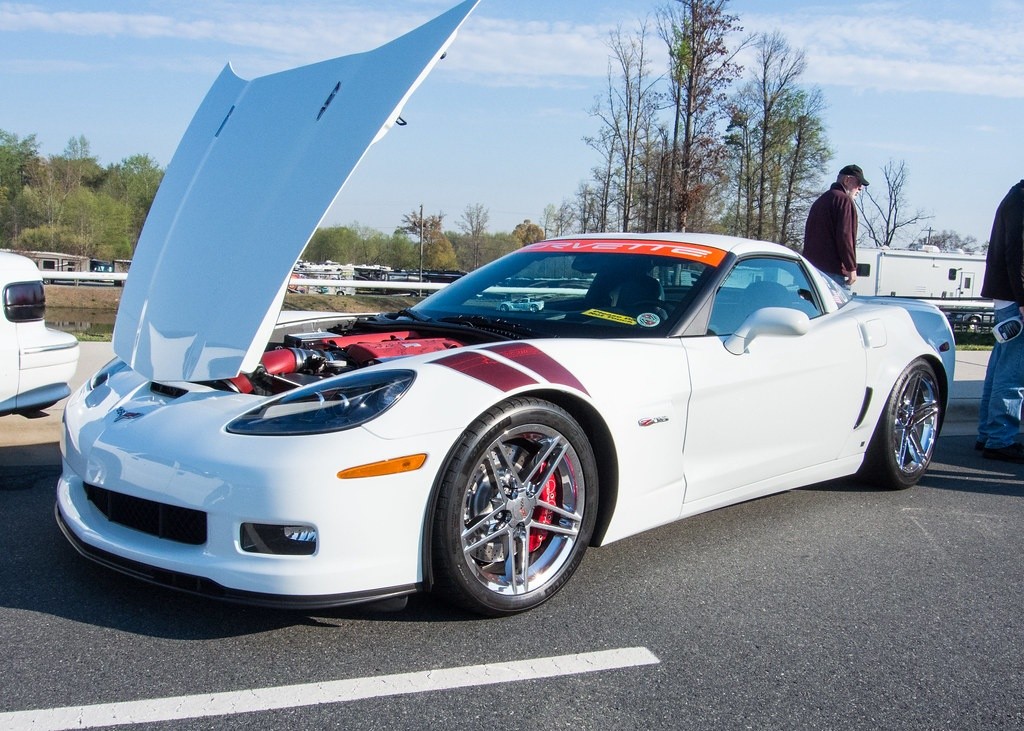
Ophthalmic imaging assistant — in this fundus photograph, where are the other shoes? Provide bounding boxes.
[975,441,1024,464]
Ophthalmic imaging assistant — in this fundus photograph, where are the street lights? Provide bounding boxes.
[419,204,423,282]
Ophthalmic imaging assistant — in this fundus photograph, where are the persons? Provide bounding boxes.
[974,179,1024,464]
[802,164,870,291]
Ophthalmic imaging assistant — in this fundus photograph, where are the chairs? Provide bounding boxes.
[616,272,669,322]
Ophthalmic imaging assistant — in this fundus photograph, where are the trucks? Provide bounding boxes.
[289,269,355,296]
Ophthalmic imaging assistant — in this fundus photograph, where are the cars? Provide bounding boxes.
[961,309,996,329]
[0,252,79,419]
[499,297,544,312]
[55,0,956,616]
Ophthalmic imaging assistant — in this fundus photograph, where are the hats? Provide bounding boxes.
[839,164,870,186]
